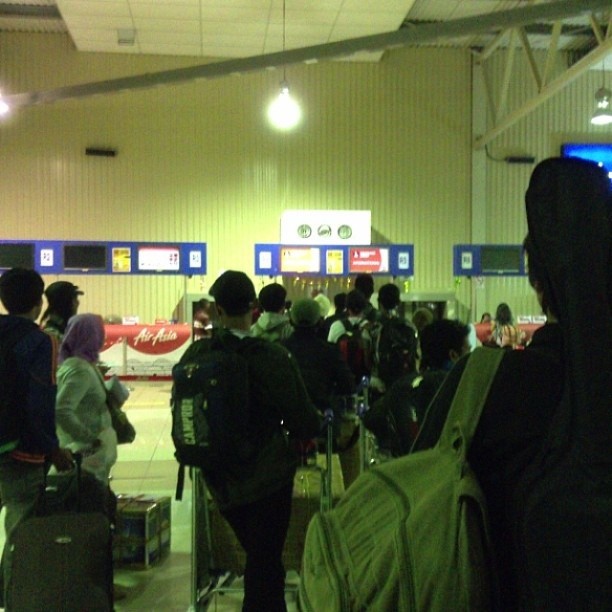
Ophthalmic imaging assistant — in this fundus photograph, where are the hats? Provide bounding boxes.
[290,299,319,327]
[209,270,255,310]
[46,281,83,297]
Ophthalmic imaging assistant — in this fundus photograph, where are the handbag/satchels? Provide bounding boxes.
[170,338,262,502]
[94,363,135,442]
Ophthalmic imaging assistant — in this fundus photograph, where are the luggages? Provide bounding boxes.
[0,451,114,611]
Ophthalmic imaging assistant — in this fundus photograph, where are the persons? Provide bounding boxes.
[246,282,295,352]
[192,298,212,337]
[170,270,325,612]
[484,302,526,348]
[411,156,611,612]
[346,274,378,330]
[39,282,83,377]
[368,318,470,454]
[327,293,380,384]
[366,284,420,377]
[54,314,135,606]
[2,268,74,590]
[274,298,345,463]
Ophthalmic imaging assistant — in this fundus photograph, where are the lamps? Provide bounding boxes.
[263,0,302,132]
[590,56,612,125]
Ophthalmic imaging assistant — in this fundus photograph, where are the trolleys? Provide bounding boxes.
[341,402,366,476]
[186,411,335,612]
[351,375,368,411]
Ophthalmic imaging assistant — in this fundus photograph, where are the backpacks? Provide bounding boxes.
[336,317,371,377]
[300,349,505,612]
